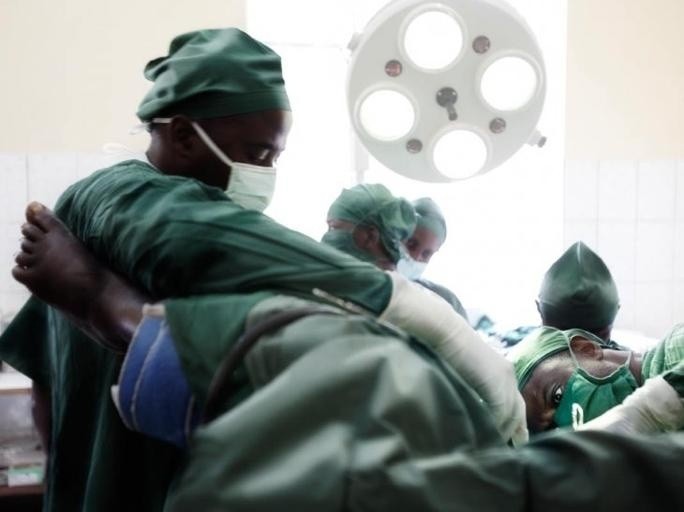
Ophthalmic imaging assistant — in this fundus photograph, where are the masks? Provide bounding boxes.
[553,340,640,433]
[186,118,276,216]
[393,242,428,286]
[319,227,374,269]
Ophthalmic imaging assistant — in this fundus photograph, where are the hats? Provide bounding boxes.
[535,240,619,331]
[411,196,446,248]
[324,181,417,245]
[133,26,291,135]
[512,326,607,394]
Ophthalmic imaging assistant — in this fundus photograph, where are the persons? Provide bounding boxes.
[0,26,684,512]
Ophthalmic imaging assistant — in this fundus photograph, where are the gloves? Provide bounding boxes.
[371,269,529,453]
